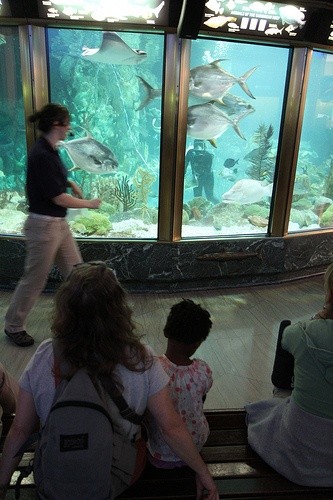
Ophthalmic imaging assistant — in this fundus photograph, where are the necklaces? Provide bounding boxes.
[322,306,333,312]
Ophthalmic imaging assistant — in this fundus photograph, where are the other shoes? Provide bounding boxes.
[4,326,35,347]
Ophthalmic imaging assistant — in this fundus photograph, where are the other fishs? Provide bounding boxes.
[136,74,162,112]
[189,57,258,104]
[80,30,147,66]
[183,93,255,150]
[221,177,274,206]
[54,124,120,179]
[219,159,240,179]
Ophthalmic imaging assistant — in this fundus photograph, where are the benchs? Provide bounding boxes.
[0,410,333,500]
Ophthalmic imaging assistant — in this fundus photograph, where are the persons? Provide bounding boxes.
[244,263,333,488]
[4,102,100,349]
[0,365,20,452]
[141,296,214,470]
[0,260,219,500]
[183,139,220,205]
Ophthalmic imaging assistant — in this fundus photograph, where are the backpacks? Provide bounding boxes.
[33,335,151,500]
